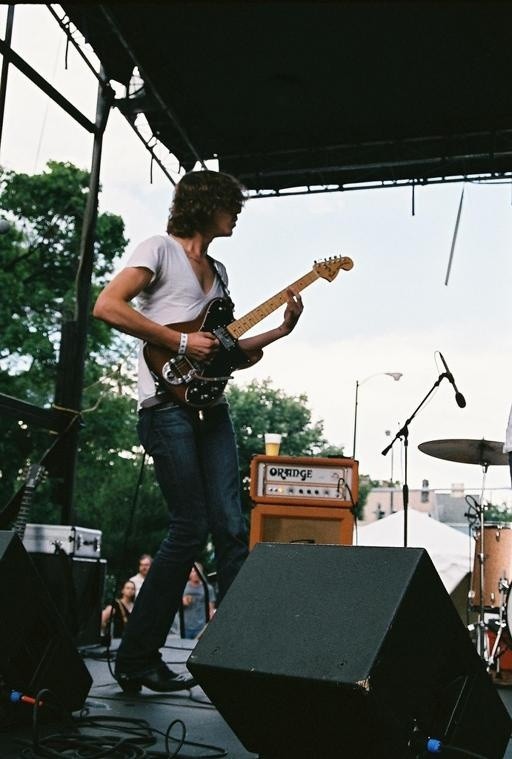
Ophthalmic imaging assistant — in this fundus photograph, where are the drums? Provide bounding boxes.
[469,521,512,614]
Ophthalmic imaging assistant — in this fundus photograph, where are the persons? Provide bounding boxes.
[171,561,220,642]
[99,580,139,639]
[90,170,305,696]
[127,552,155,600]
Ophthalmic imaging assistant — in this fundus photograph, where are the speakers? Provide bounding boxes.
[249,504,354,552]
[0,530,94,730]
[185,541,512,759]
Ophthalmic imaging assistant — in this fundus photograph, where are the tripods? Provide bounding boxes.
[461,464,512,669]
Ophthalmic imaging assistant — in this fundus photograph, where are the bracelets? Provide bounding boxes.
[177,332,189,355]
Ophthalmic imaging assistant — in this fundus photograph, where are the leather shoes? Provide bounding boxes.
[117,665,199,693]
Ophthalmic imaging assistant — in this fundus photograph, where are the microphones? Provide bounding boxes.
[439,352,466,409]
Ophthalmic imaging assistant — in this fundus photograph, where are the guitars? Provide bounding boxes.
[143,255,353,408]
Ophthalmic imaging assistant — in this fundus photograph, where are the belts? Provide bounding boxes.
[140,392,174,409]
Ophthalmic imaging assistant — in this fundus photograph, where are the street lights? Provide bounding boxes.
[352,372,402,458]
[385,431,394,513]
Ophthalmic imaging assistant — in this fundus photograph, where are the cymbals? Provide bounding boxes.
[418,439,511,465]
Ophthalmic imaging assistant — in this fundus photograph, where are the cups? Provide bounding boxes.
[264,433,282,456]
